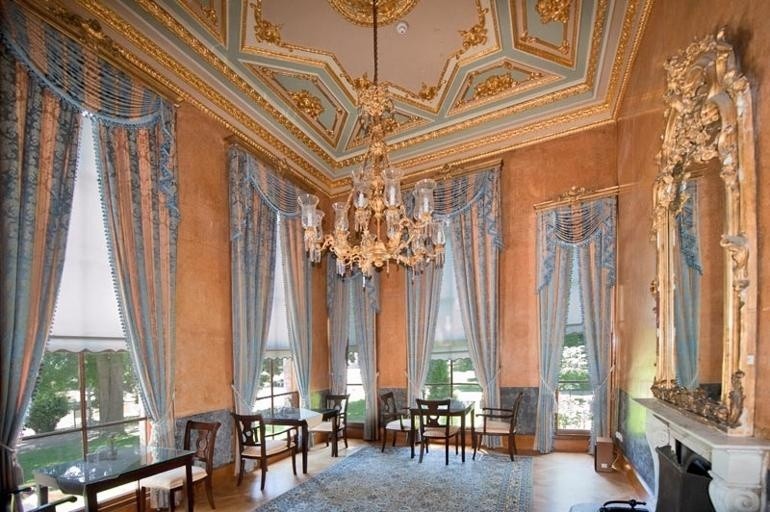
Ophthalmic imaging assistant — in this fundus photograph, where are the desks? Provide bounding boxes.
[407,400,476,462]
[253,406,338,474]
[32,445,199,512]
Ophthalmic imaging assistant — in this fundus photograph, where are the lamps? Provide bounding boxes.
[297,0,445,287]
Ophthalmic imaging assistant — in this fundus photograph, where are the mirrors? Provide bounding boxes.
[651,24,757,439]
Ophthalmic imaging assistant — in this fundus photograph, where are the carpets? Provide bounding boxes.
[250,444,534,511]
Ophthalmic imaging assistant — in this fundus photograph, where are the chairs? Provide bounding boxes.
[0,444,77,512]
[325,394,348,457]
[139,419,221,512]
[472,393,523,462]
[380,391,459,466]
[231,412,298,489]
